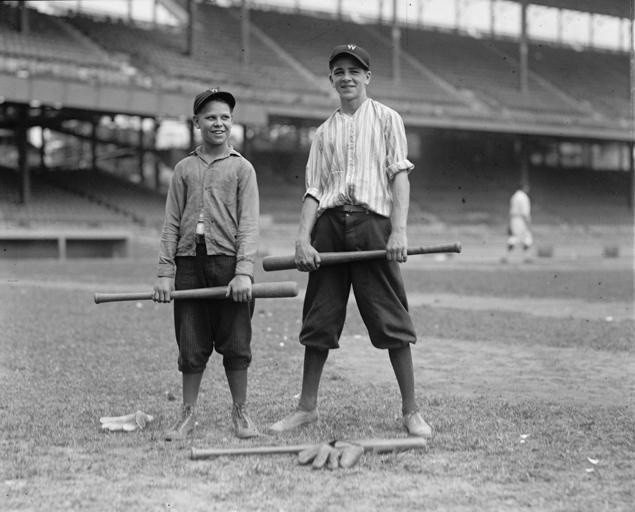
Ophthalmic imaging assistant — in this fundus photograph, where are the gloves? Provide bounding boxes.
[99,411,155,431]
[299,440,365,470]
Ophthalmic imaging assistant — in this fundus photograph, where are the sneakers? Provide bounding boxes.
[399,408,431,436]
[230,406,260,439]
[268,406,320,432]
[163,406,199,442]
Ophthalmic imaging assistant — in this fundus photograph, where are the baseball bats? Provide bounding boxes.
[191,435,427,458]
[263,240,462,271]
[92,280,299,304]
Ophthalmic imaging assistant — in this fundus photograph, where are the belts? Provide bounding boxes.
[329,205,372,217]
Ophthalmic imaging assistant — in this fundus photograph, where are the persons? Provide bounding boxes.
[270,45,435,438]
[152,88,259,442]
[501,182,534,265]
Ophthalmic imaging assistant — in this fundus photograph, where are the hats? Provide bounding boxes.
[327,44,372,72]
[192,88,235,115]
